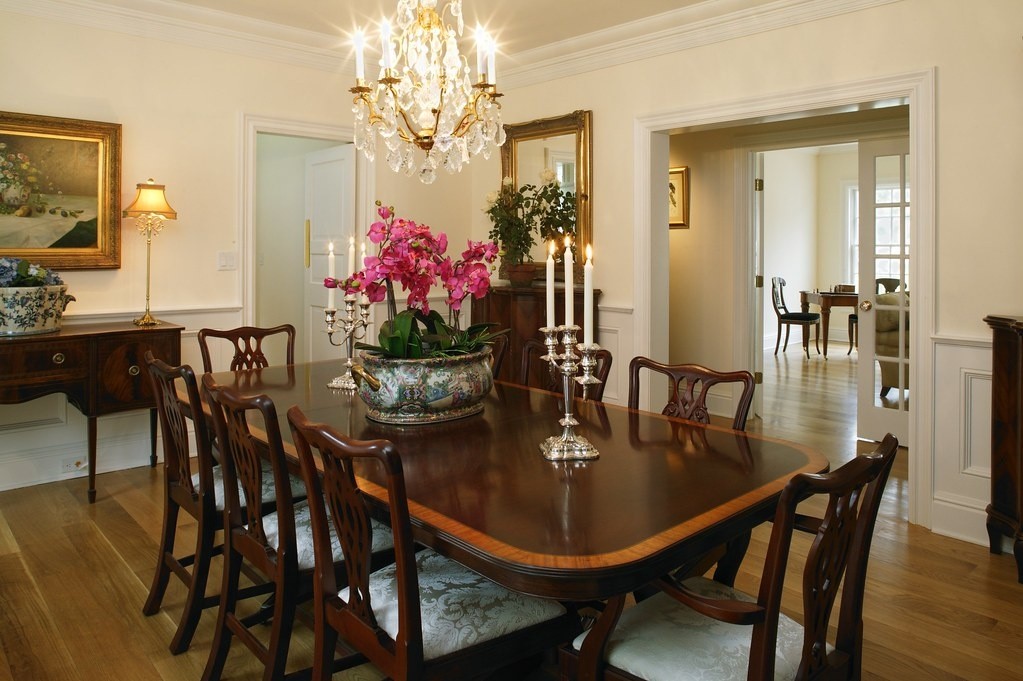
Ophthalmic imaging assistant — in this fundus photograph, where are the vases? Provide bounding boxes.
[0,284,77,336]
[350,344,495,425]
[506,261,536,284]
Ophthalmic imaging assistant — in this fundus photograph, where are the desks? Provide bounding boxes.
[982,313,1023,591]
[799,291,858,361]
[0,318,185,504]
[470,285,603,393]
[173,354,830,681]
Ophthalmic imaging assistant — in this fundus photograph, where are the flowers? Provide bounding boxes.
[538,190,578,264]
[482,170,563,265]
[323,199,511,363]
[0,256,64,288]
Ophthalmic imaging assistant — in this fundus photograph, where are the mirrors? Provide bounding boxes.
[497,108,593,279]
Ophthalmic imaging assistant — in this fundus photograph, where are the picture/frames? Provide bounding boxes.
[669,166,689,229]
[0,110,123,271]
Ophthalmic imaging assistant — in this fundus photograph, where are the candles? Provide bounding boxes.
[584,243,594,346]
[361,243,369,302]
[347,236,355,299]
[546,240,555,328]
[564,237,573,328]
[327,243,335,309]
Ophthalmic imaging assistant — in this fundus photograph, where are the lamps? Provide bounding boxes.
[122,178,177,326]
[349,0,508,184]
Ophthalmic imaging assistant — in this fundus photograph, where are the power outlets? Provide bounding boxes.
[61,455,85,474]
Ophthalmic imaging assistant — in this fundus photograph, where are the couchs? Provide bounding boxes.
[874,289,911,397]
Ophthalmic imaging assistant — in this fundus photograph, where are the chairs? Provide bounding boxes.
[471,333,509,378]
[287,405,571,681]
[771,276,821,360]
[558,430,899,681]
[196,324,295,467]
[521,338,613,403]
[139,350,327,657]
[627,356,757,431]
[202,372,426,681]
[847,278,907,355]
